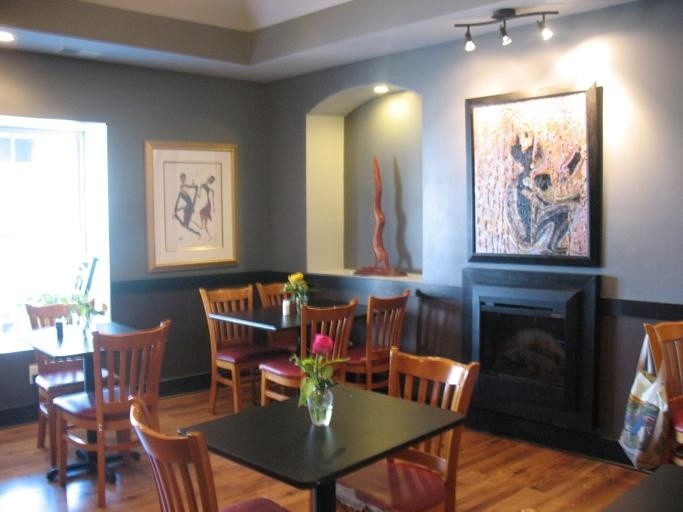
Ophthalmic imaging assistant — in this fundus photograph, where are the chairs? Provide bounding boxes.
[51,320,173,491]
[258,300,361,407]
[198,283,296,416]
[255,282,311,355]
[345,288,411,398]
[335,346,481,511]
[126,391,292,512]
[25,298,122,468]
[641,320,683,467]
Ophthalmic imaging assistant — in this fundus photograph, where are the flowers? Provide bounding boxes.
[283,271,312,317]
[74,295,108,341]
[288,334,352,426]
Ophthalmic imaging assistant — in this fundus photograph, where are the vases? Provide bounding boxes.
[295,294,308,316]
[306,385,334,426]
[82,318,96,341]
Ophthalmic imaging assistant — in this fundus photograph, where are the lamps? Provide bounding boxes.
[453,7,560,53]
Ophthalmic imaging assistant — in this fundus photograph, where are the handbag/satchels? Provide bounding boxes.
[618,328,671,472]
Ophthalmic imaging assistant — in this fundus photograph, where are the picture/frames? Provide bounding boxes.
[463,80,605,266]
[143,139,242,274]
